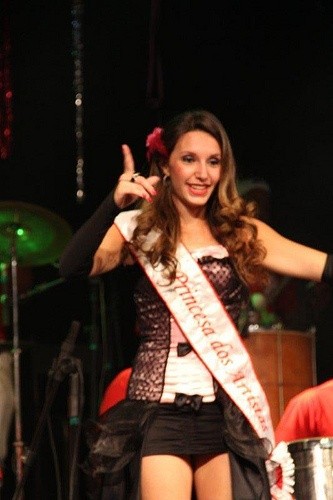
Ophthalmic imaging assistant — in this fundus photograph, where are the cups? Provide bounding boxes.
[287,436,333,500]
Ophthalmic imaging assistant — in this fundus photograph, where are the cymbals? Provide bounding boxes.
[0,198,73,267]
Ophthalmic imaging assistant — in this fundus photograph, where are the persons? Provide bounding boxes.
[60,110,333,500]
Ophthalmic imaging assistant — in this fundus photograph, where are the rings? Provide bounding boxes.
[131,172,140,182]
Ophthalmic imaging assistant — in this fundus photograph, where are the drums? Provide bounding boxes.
[242,323,318,432]
[288,436,333,500]
